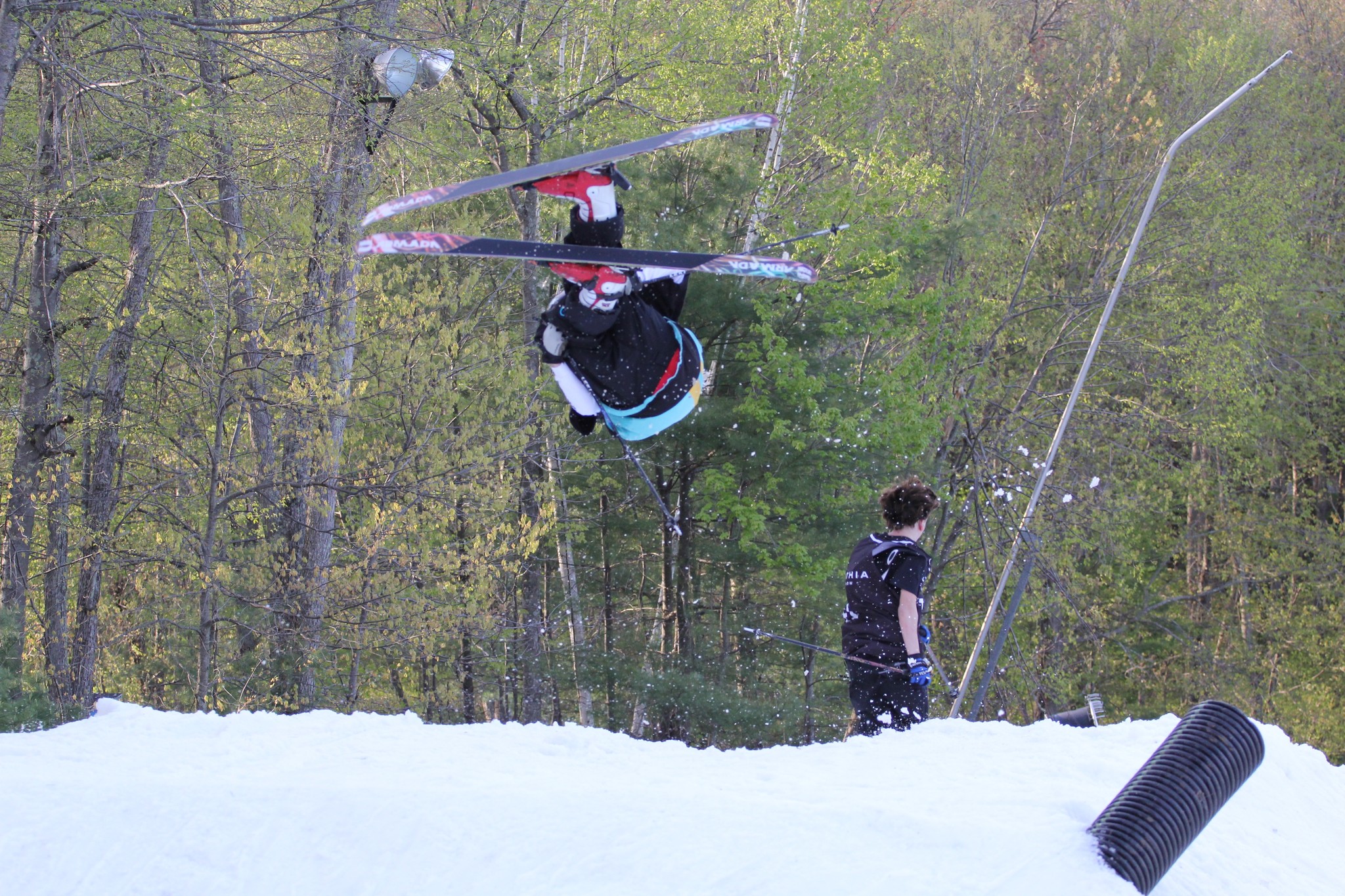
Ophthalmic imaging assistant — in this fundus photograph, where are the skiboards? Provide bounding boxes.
[354,113,819,285]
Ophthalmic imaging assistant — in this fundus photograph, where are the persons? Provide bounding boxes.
[840,474,941,737]
[513,159,705,442]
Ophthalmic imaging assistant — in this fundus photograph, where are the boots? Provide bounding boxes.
[546,262,626,313]
[513,163,618,223]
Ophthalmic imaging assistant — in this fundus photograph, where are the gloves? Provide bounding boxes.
[908,653,931,687]
[918,625,931,645]
[531,294,575,358]
